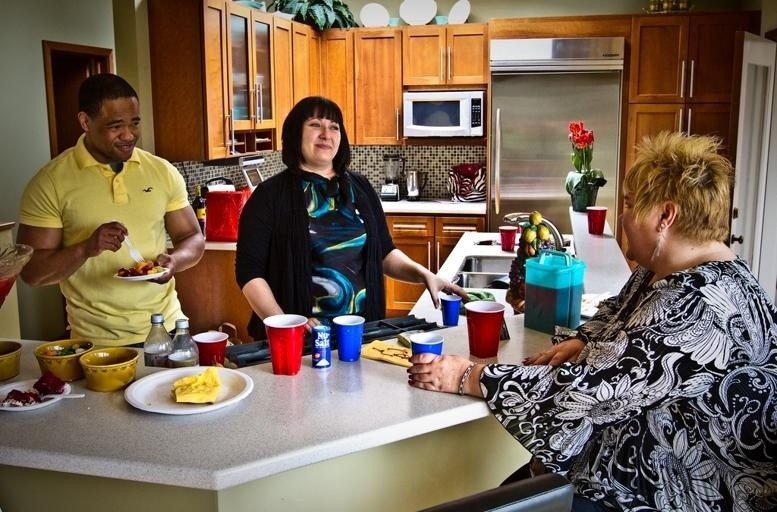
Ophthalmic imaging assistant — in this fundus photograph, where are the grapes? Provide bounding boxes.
[141,265,152,274]
[509,235,553,299]
[118,268,140,276]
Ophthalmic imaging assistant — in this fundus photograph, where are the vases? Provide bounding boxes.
[570,183,599,213]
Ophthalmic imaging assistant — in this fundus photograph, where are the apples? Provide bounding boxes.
[529,210,543,225]
[524,227,537,243]
[538,223,549,240]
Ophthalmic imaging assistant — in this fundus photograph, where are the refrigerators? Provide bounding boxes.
[488,37,624,239]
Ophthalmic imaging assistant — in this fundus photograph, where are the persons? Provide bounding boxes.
[16,72,206,346]
[235,95,470,342]
[407,130,777,512]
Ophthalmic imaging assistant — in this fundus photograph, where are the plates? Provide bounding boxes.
[399,0,439,27]
[0,380,72,411]
[113,263,169,282]
[231,73,268,119]
[124,366,252,417]
[359,3,390,28]
[448,1,471,25]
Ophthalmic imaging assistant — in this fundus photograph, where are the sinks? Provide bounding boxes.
[453,273,511,288]
[461,255,518,272]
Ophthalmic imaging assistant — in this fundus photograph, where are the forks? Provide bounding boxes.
[111,217,147,264]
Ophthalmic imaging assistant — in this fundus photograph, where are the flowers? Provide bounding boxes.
[565,120,608,195]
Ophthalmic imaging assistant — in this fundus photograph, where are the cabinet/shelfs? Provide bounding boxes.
[627,15,753,103]
[621,102,740,269]
[384,215,487,318]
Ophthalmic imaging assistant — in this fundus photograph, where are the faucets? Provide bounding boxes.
[503,212,563,251]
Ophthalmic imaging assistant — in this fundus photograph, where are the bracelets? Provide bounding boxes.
[459,362,478,396]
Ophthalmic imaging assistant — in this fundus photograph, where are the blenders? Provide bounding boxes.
[377,154,406,200]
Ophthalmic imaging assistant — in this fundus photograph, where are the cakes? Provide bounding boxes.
[34,369,66,402]
[2,389,41,408]
[170,366,221,404]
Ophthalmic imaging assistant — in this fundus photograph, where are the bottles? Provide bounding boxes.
[191,183,207,240]
[312,325,332,368]
[167,316,198,365]
[143,314,173,365]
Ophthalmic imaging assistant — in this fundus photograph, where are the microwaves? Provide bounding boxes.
[402,92,484,137]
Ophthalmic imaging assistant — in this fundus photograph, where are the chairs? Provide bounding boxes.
[417,472,575,512]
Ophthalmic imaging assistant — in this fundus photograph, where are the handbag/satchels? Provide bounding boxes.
[446,164,486,202]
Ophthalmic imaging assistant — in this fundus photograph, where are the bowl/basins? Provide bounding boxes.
[33,338,94,382]
[78,347,140,392]
[0,341,22,379]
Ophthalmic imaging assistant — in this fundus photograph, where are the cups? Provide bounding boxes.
[409,332,444,356]
[332,314,366,361]
[440,294,461,327]
[586,206,607,235]
[464,300,505,358]
[192,331,229,365]
[261,314,308,376]
[405,170,429,202]
[499,225,516,251]
